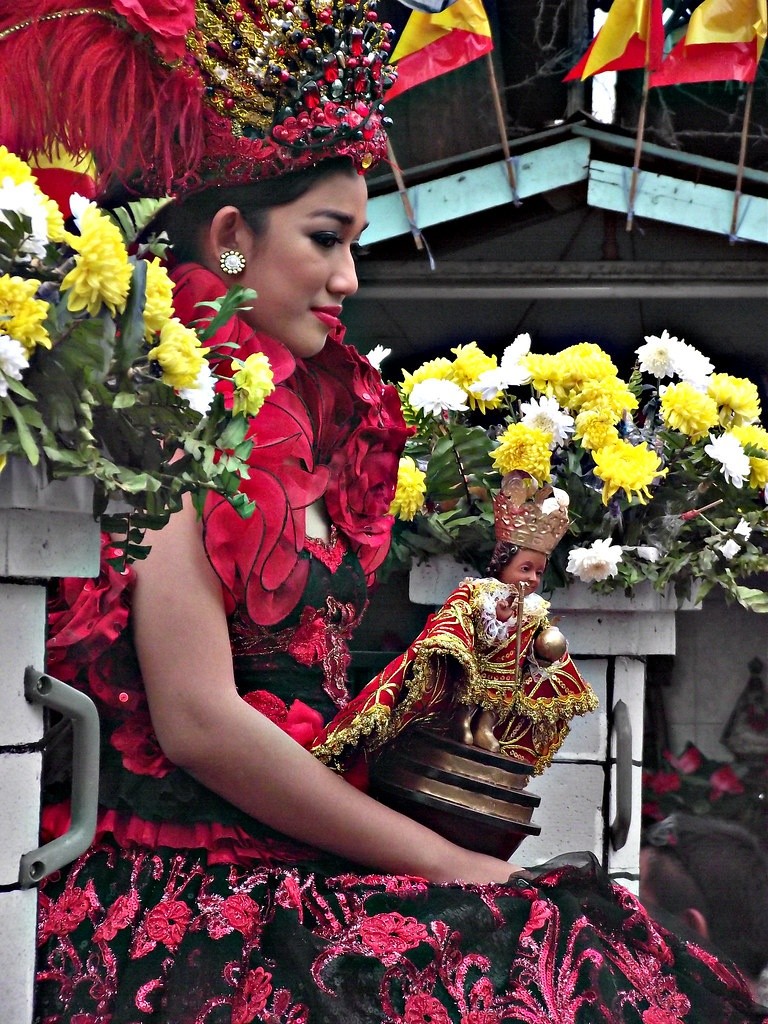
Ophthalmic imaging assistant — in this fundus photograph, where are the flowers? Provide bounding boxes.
[0,144,768,616]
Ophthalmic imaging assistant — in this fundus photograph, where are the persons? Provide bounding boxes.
[450,469,569,752]
[1,2,767,1022]
